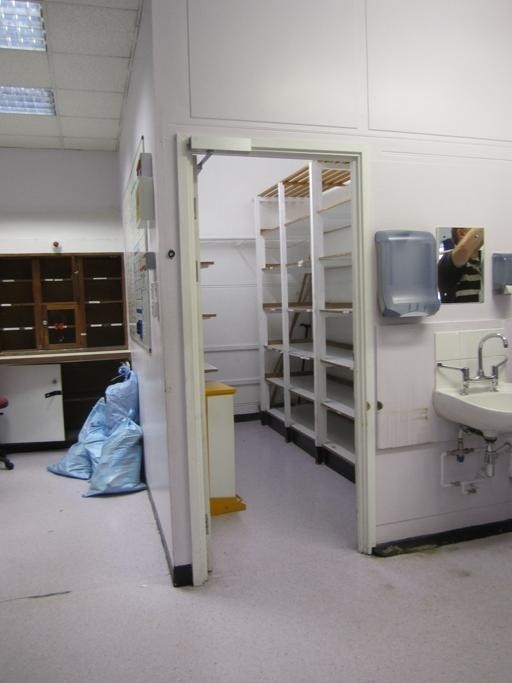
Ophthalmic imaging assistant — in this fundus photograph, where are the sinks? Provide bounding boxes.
[432,383,512,432]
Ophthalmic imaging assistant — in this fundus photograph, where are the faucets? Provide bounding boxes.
[479,333,508,372]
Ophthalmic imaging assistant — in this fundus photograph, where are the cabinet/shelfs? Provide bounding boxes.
[0,251,131,453]
[252,156,355,486]
[198,236,304,419]
